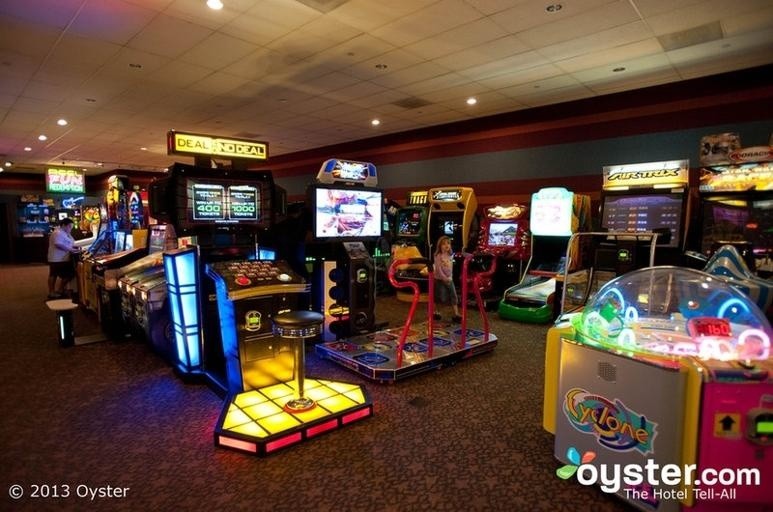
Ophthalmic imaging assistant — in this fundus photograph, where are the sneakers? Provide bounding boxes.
[452,315,461,321]
[49,290,67,298]
[433,314,441,320]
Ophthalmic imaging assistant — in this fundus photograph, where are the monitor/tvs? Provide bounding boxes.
[600,188,686,249]
[430,212,463,252]
[177,171,271,231]
[85,220,165,253]
[699,191,773,251]
[399,209,422,233]
[488,223,518,246]
[316,188,381,237]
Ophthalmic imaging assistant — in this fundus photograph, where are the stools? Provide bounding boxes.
[272,311,325,414]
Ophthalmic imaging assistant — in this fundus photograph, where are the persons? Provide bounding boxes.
[71,219,92,241]
[47,219,81,297]
[433,236,462,323]
